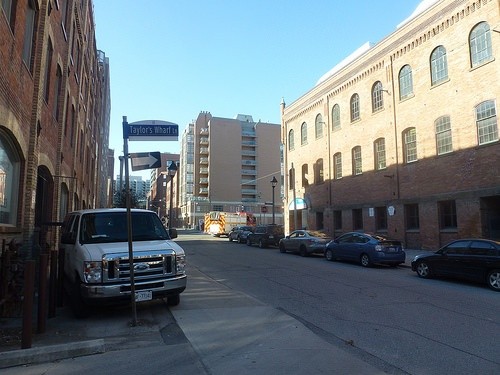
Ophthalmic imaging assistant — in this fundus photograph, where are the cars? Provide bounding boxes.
[411,238,500,292]
[279,230,328,257]
[229,226,251,244]
[324,231,406,268]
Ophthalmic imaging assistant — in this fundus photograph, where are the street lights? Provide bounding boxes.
[169,161,178,235]
[269,176,278,225]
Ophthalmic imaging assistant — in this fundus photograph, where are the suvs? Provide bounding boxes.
[247,225,285,248]
[58,208,188,320]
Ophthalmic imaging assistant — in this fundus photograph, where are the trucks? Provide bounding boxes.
[204,212,256,237]
